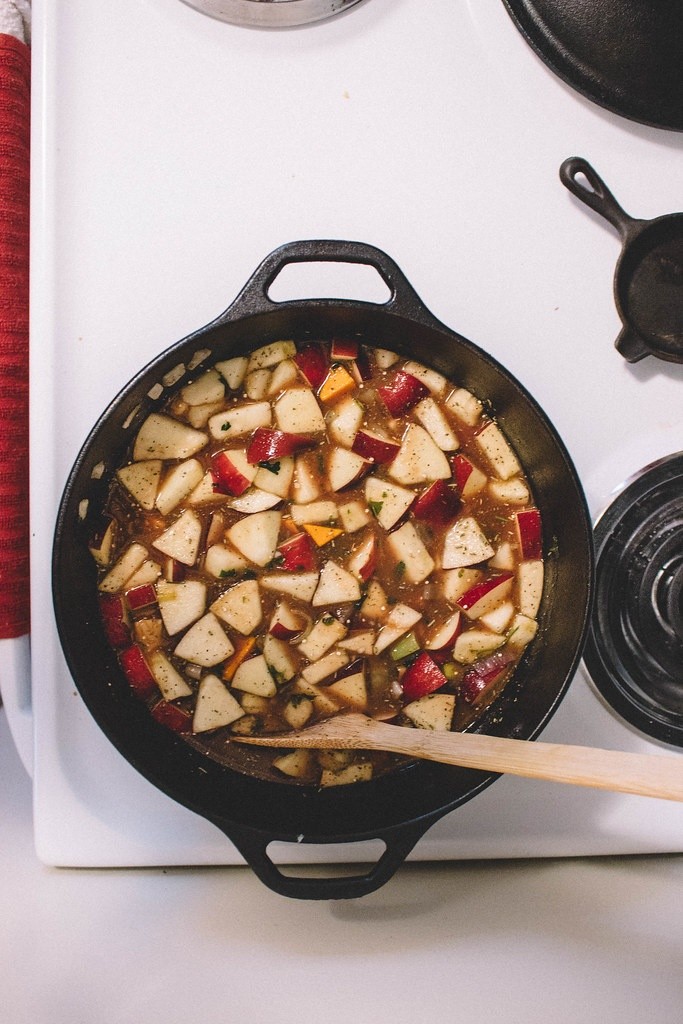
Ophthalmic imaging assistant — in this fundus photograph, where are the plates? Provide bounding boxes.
[181,0,361,28]
[501,0,683,132]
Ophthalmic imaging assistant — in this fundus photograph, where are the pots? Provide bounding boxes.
[52,238,592,901]
[559,156,683,365]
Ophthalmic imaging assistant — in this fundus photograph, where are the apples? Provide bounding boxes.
[100,340,544,785]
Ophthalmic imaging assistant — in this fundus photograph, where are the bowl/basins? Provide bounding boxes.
[580,450,683,755]
